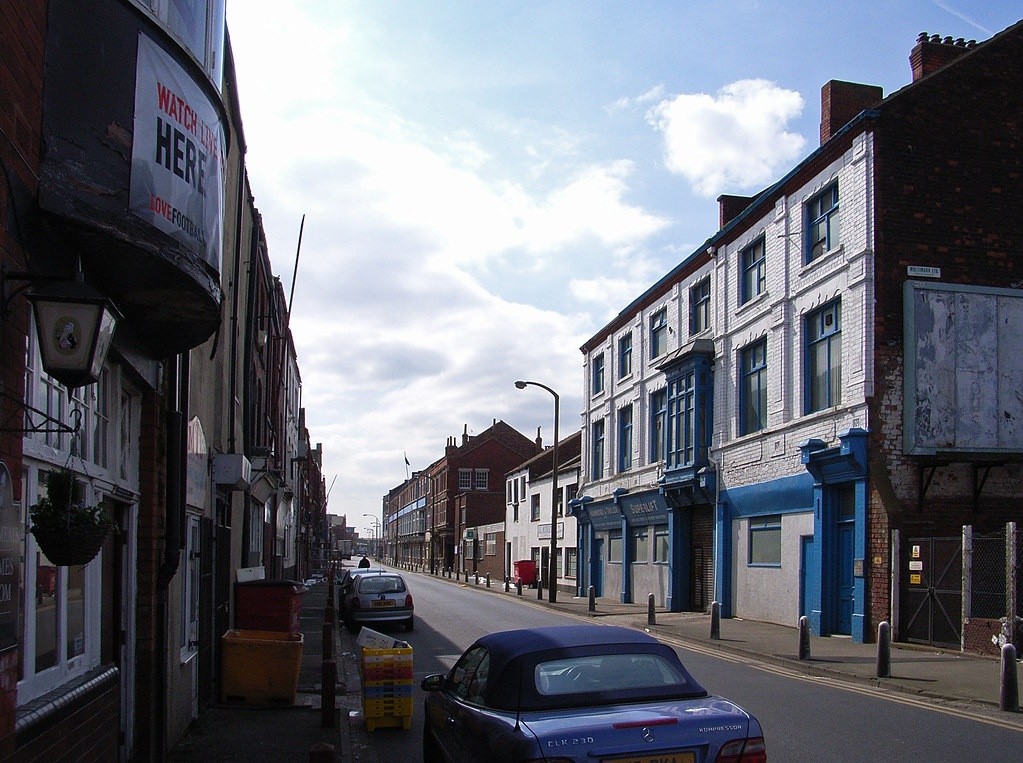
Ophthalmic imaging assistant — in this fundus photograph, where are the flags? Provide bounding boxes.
[405,457,410,465]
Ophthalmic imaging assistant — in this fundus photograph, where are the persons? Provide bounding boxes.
[445,549,452,571]
[358,555,370,568]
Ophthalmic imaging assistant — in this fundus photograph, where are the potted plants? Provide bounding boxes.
[27,466,118,566]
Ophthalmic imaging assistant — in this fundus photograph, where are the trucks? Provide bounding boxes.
[338,540,352,560]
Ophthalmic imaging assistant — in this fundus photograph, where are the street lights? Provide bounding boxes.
[514,381,561,605]
[385,502,398,566]
[363,521,381,557]
[363,514,381,542]
[414,473,435,574]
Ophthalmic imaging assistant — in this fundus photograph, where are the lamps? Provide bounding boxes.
[0,254,126,404]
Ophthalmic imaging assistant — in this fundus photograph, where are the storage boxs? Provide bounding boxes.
[361,641,415,731]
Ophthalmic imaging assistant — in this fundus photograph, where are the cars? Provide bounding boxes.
[420,626,767,763]
[337,568,395,599]
[340,573,415,633]
